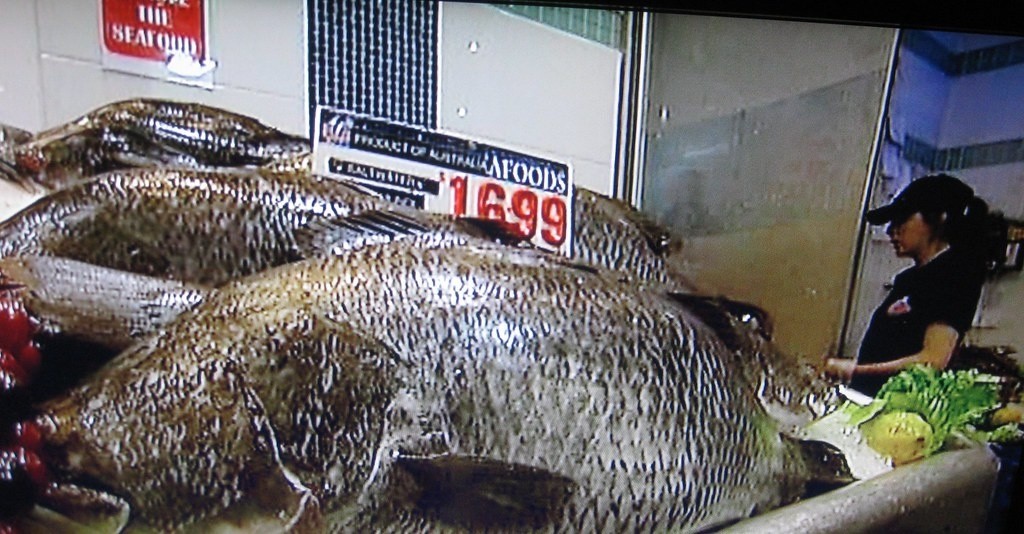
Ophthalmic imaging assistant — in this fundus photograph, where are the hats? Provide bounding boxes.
[864,170,973,227]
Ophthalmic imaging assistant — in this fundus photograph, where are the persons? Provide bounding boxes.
[823,173,995,400]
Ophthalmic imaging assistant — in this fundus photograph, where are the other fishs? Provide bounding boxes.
[3,97,863,534]
